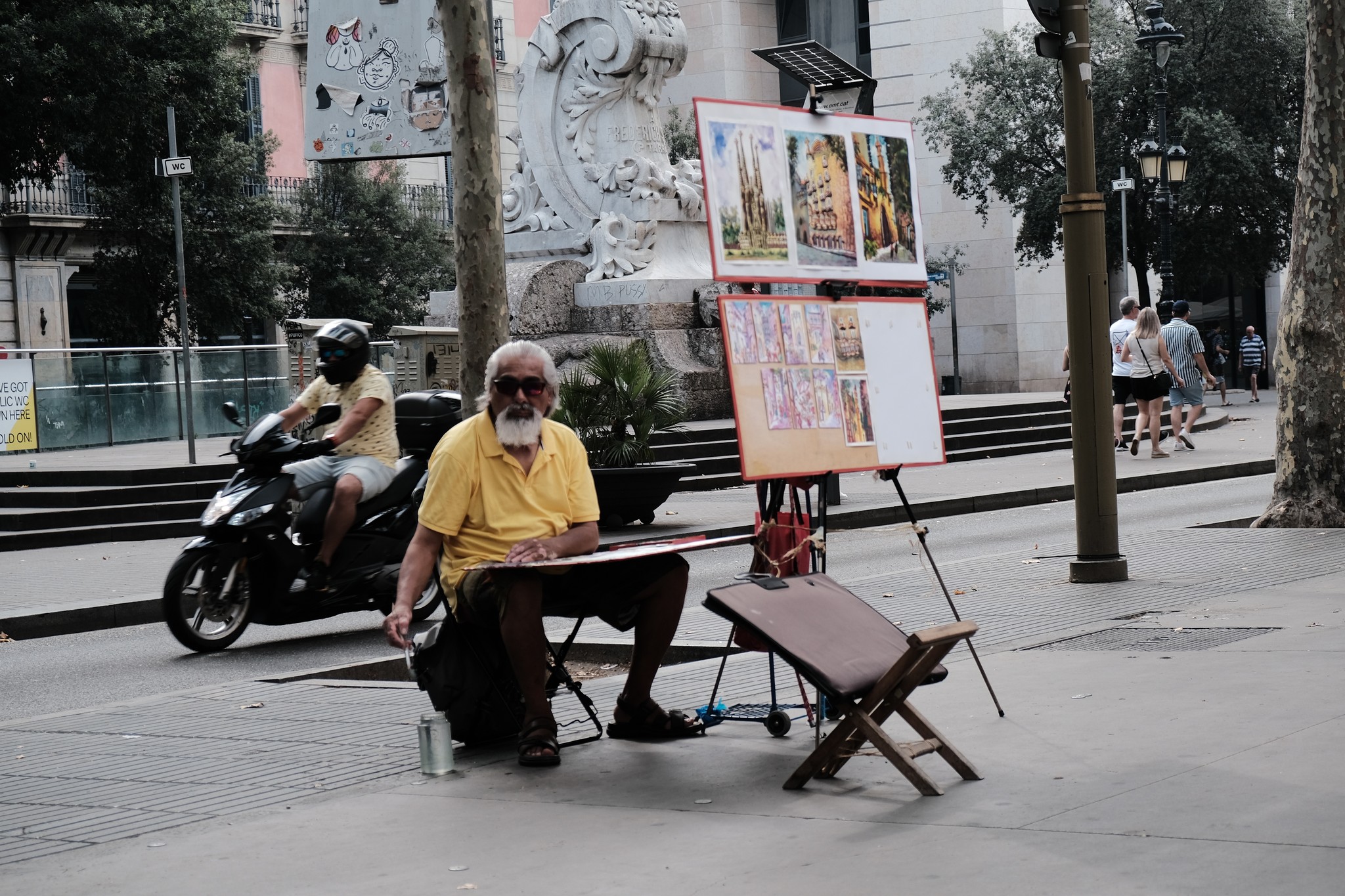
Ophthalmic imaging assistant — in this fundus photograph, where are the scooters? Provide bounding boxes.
[164,388,462,654]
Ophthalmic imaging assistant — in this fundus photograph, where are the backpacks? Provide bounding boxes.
[1203,333,1219,366]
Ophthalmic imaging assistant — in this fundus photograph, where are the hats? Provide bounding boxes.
[1172,300,1192,314]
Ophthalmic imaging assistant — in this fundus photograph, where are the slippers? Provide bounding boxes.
[1221,401,1233,406]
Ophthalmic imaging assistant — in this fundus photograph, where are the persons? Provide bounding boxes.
[382,341,704,764]
[1202,324,1233,406]
[1161,301,1216,451]
[1110,296,1169,452]
[276,319,399,598]
[754,479,783,512]
[1238,326,1266,403]
[824,491,847,500]
[1062,342,1113,460]
[1121,307,1184,459]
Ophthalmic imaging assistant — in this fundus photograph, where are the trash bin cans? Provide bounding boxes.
[941,375,962,396]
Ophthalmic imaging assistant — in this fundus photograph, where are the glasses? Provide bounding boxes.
[1134,306,1142,310]
[321,348,346,361]
[491,375,548,397]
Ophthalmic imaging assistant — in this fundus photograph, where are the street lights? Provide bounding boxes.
[1133,0,1191,325]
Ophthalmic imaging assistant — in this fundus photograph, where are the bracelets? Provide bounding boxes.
[1263,362,1266,364]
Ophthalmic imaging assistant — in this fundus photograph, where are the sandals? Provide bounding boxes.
[517,716,561,767]
[606,693,701,741]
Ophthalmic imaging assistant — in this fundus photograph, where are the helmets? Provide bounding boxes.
[315,323,371,385]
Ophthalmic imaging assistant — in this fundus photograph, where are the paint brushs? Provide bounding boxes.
[392,602,412,671]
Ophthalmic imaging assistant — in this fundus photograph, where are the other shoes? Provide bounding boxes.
[1114,438,1120,449]
[1174,441,1188,451]
[839,491,848,500]
[1116,439,1129,451]
[1158,431,1169,446]
[1151,448,1170,458]
[1249,399,1260,403]
[306,560,333,592]
[1179,428,1196,449]
[1130,437,1140,456]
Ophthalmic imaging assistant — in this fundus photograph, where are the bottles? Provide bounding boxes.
[416,711,454,774]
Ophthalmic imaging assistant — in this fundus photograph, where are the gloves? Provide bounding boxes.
[301,439,334,460]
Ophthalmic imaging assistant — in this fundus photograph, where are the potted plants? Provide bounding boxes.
[551,333,695,531]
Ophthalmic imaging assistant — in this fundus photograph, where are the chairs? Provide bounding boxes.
[418,539,610,754]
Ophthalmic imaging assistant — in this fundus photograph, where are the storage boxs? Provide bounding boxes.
[394,383,469,460]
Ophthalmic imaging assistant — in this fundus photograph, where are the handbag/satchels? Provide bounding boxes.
[749,484,810,578]
[1153,372,1174,390]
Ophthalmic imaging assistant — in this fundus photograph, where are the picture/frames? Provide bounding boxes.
[714,289,948,479]
[688,95,931,293]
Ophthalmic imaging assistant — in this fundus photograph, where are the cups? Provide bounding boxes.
[1207,379,1214,390]
